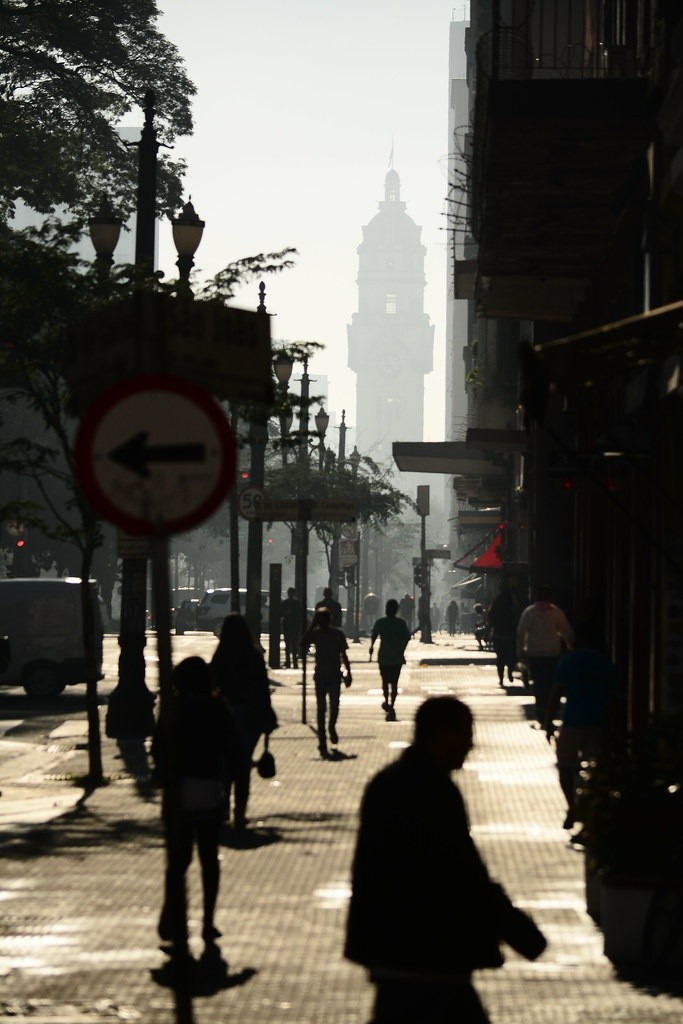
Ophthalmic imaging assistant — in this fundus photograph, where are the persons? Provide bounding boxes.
[399,594,415,631]
[302,606,352,743]
[446,601,458,634]
[473,603,489,648]
[370,599,411,721]
[429,602,441,633]
[151,614,275,942]
[490,585,619,828]
[342,696,547,1024]
[315,588,342,626]
[280,588,302,669]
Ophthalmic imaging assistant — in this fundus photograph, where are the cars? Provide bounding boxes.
[146,587,200,635]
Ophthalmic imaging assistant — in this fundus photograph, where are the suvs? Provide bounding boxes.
[197,585,316,640]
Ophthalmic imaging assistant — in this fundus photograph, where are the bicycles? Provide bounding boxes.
[439,620,466,636]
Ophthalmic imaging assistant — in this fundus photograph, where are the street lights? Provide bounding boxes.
[279,355,331,620]
[324,409,361,602]
[236,280,298,640]
[88,108,207,740]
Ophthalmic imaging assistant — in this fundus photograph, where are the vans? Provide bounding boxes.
[0,576,105,702]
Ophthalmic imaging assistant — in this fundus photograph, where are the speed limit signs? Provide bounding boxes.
[238,487,267,521]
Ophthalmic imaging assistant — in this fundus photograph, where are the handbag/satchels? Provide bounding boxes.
[258,734,276,778]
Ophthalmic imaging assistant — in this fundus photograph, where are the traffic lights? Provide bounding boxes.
[15,538,27,549]
[239,468,252,483]
